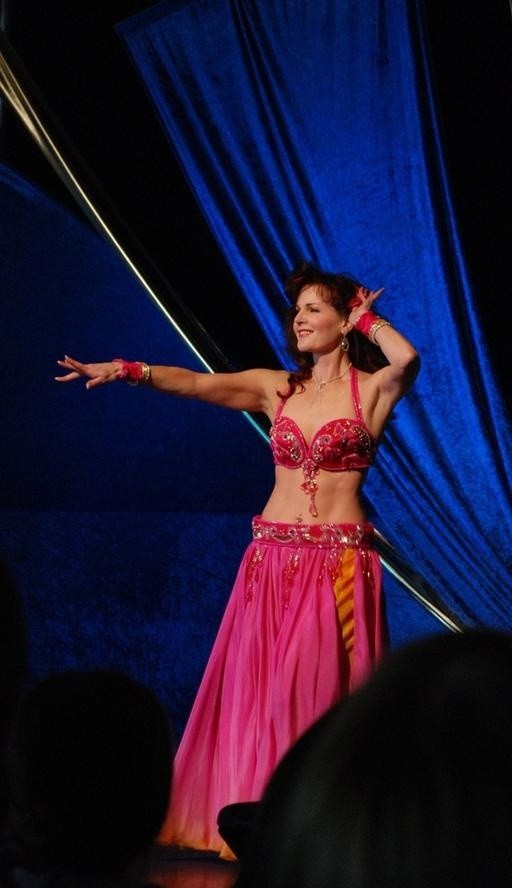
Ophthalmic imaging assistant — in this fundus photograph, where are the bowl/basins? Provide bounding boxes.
[309,362,354,392]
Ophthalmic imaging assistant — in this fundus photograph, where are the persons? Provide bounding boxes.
[52,257,422,866]
[2,667,177,886]
[213,618,512,888]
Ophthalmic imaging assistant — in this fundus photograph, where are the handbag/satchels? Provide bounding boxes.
[354,310,392,347]
[112,359,150,387]
